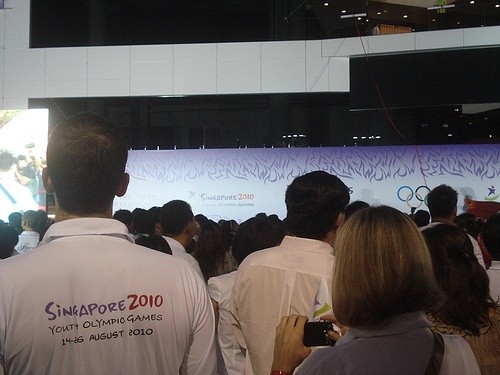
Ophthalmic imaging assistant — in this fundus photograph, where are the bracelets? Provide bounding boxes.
[270,370,293,375]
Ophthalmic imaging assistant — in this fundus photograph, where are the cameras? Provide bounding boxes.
[303,319,336,347]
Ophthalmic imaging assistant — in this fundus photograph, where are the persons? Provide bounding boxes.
[0,111,217,375]
[0,170,500,375]
[0,152,46,215]
